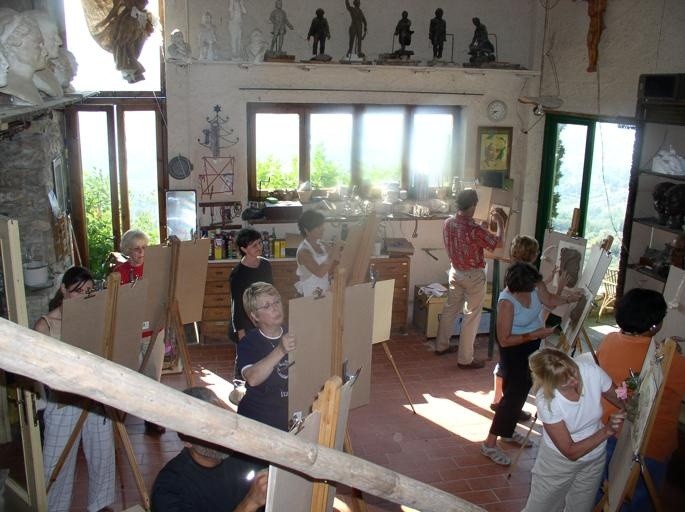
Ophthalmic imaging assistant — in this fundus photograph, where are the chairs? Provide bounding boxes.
[596,266,621,322]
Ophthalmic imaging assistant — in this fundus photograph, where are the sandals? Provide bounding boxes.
[501,432,533,446]
[479,443,511,466]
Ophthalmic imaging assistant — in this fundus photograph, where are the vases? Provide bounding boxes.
[23,262,49,289]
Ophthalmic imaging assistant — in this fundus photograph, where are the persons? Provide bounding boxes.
[231,228,278,404]
[478,265,558,465]
[169,0,504,67]
[522,348,637,512]
[582,0,607,73]
[111,224,170,439]
[236,280,306,440]
[481,134,508,172]
[488,234,583,417]
[436,188,505,367]
[1,9,78,104]
[649,141,685,177]
[645,182,683,223]
[293,211,342,304]
[32,267,117,512]
[141,386,277,511]
[593,288,685,512]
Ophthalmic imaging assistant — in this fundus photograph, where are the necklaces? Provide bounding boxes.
[263,326,281,349]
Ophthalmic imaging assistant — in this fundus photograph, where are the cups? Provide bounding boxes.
[249,174,462,217]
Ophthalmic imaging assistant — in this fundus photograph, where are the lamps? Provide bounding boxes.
[516,1,566,117]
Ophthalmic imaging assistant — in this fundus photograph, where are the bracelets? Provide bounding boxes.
[566,296,571,303]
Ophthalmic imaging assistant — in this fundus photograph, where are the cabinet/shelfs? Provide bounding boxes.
[613,71,685,333]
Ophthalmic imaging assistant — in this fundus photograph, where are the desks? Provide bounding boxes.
[200,253,411,347]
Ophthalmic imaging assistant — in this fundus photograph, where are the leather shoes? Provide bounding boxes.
[436,346,457,355]
[492,403,531,420]
[457,359,485,369]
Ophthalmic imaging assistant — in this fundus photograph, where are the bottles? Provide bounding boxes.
[200,227,287,260]
[666,238,677,260]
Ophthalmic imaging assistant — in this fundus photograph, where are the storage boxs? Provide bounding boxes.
[413,283,451,339]
[453,306,497,338]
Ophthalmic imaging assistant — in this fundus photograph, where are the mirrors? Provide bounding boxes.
[164,190,198,242]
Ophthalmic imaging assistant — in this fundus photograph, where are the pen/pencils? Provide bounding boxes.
[552,324,559,330]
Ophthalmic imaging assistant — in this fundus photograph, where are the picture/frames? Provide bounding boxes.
[51,156,70,217]
[475,126,513,177]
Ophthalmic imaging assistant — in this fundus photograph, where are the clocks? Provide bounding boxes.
[486,99,507,123]
